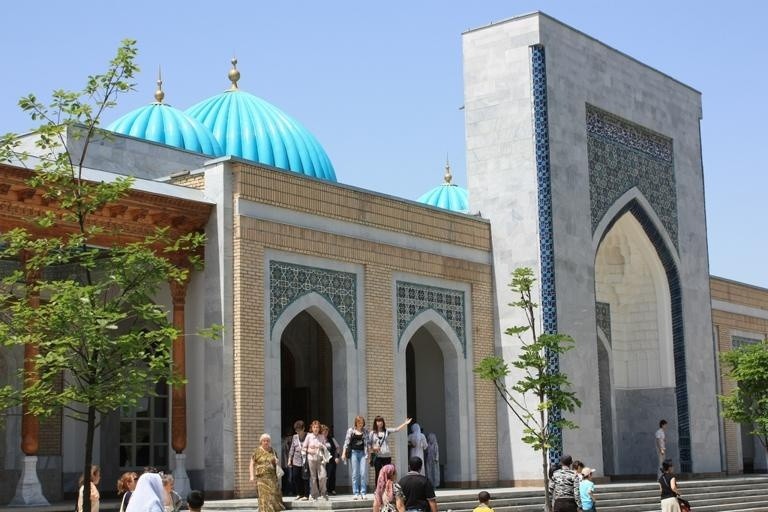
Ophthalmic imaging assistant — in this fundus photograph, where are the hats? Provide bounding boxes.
[561,455,572,465]
[580,468,595,478]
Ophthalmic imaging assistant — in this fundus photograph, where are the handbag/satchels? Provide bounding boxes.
[301,463,309,480]
[370,455,377,467]
[275,464,284,480]
[346,448,351,459]
[677,498,690,512]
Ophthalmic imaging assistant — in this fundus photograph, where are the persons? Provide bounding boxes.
[549,454,583,512]
[579,466,597,512]
[75,465,204,512]
[246,413,494,512]
[654,419,668,481]
[572,460,585,483]
[658,458,681,512]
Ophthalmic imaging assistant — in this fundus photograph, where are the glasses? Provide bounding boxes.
[132,478,138,481]
